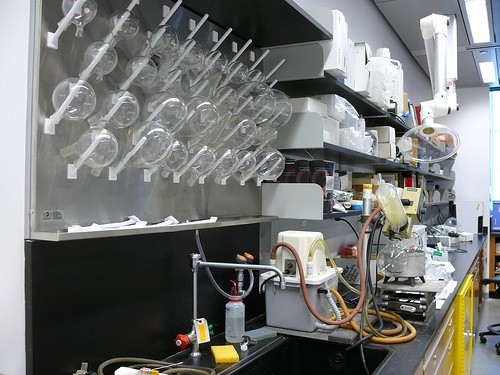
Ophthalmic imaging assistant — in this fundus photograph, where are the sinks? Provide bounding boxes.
[208,330,398,375]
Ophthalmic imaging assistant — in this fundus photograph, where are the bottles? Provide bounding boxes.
[225,297,245,343]
[375,179,407,227]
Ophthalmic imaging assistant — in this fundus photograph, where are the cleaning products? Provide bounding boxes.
[225,279,246,344]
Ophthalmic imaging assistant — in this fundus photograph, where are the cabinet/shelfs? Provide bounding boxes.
[152,232,487,375]
[261,40,455,220]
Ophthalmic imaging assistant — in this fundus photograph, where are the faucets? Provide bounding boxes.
[173,252,287,358]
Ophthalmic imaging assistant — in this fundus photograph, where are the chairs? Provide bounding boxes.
[479,275,500,355]
